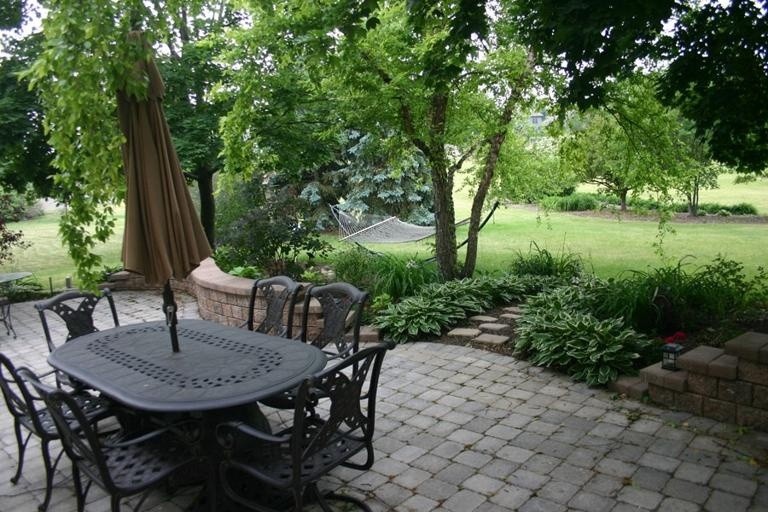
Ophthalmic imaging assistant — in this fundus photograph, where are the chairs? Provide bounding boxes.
[1,352,128,512]
[16,365,209,511]
[34,287,120,433]
[267,282,370,428]
[239,276,305,338]
[214,339,398,512]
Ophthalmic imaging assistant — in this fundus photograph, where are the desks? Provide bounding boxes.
[47,318,327,507]
[0,271,33,339]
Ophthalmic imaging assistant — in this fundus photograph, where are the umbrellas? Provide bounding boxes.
[115,30,214,353]
